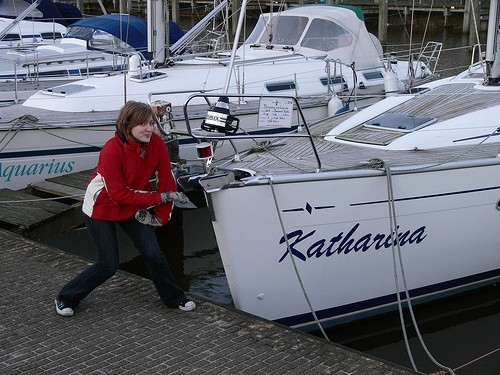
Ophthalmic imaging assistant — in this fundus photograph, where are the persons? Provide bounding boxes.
[55,101,196,316]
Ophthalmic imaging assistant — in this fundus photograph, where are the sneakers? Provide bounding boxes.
[54,296,73,317]
[179,297,196,311]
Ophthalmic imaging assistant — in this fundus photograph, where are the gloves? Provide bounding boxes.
[162,192,189,205]
[135,208,164,226]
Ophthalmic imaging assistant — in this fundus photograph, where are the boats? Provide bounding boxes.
[146,0,500,334]
[0,0,444,195]
[0,0,230,79]
